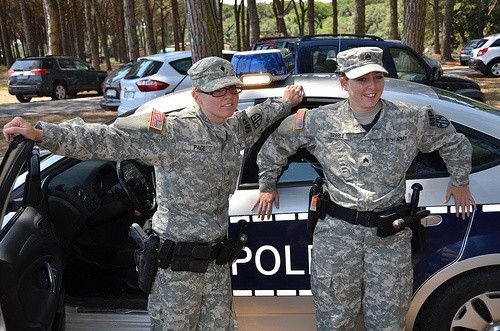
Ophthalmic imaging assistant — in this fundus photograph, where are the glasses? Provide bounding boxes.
[197,85,243,97]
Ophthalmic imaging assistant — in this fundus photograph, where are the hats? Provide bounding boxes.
[337,46,389,79]
[187,56,242,93]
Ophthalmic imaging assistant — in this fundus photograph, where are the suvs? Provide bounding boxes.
[468,33,500,78]
[6,54,109,103]
[116,49,240,118]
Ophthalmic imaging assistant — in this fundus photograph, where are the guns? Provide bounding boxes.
[309,177,323,218]
[128,223,160,294]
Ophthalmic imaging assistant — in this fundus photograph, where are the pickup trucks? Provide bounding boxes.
[293,33,488,104]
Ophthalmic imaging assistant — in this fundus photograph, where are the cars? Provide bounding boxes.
[99,61,139,112]
[381,40,444,77]
[245,35,304,58]
[460,39,482,66]
[0,49,500,331]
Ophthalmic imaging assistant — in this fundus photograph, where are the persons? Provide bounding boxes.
[252,45,477,328]
[3,56,305,330]
